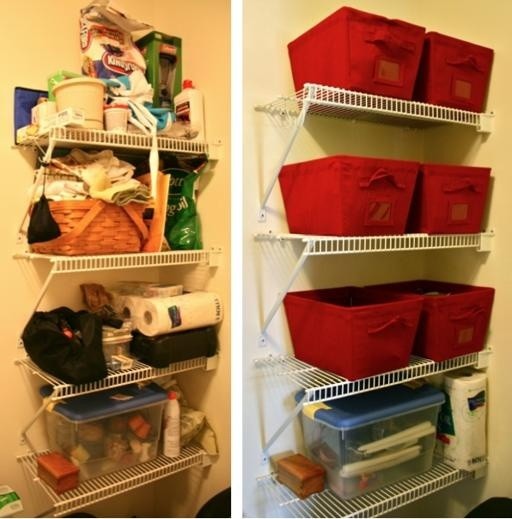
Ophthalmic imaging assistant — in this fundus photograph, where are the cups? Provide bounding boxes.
[104,102,129,134]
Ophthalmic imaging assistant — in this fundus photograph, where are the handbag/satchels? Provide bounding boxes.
[22,306,123,385]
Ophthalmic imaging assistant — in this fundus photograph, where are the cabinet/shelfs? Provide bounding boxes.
[249,85,492,518]
[15,121,218,517]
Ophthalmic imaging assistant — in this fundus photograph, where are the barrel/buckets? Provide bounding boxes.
[50,77,107,129]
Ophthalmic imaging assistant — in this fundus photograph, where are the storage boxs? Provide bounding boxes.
[276,153,492,235]
[40,384,170,482]
[292,388,446,503]
[281,277,496,381]
[286,6,495,109]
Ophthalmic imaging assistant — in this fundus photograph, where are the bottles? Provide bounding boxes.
[174,78,204,147]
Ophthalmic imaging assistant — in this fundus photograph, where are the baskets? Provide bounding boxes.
[29,198,149,255]
